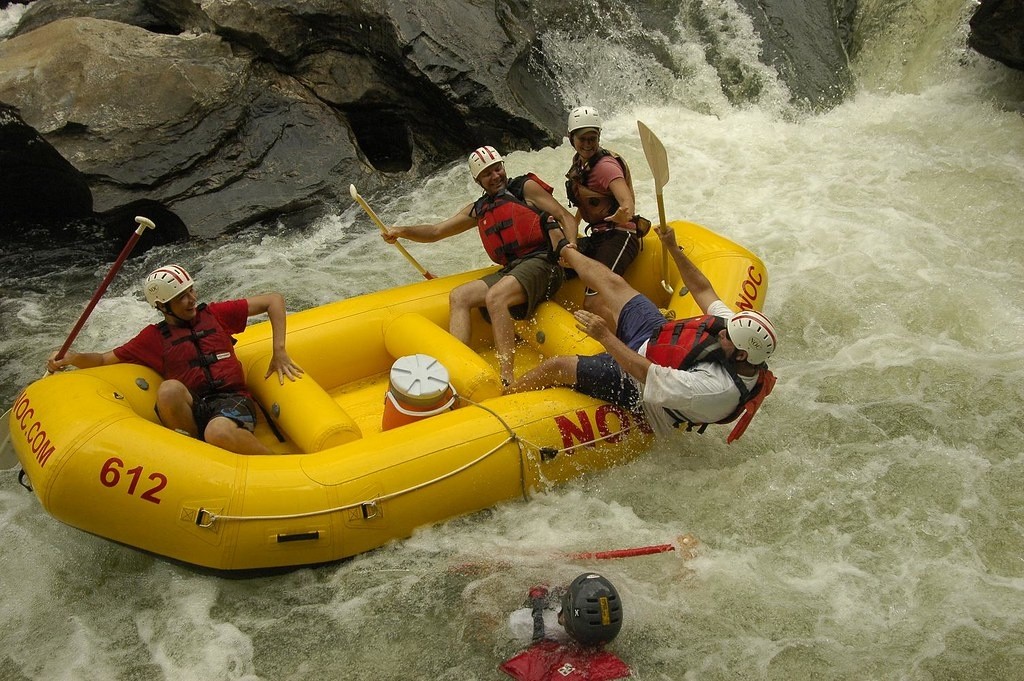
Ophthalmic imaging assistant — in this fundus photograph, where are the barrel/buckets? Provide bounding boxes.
[381,355,458,433]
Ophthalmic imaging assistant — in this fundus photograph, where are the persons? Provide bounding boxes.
[510,573,623,647]
[381,146,578,388]
[47,264,305,456]
[564,106,640,334]
[503,211,777,446]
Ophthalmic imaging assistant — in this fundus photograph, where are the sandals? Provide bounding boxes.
[539,212,570,264]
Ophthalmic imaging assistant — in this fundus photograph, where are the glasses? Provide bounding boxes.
[725,327,731,341]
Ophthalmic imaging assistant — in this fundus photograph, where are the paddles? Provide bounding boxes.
[350,182,439,280]
[0,214,157,472]
[571,534,697,559]
[637,120,675,295]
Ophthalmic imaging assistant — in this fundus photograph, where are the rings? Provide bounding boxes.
[585,323,588,327]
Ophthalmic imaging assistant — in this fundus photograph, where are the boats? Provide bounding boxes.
[9,221,770,579]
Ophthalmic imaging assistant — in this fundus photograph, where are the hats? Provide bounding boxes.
[573,128,599,137]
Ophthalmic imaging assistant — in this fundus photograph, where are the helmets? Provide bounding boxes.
[727,309,777,365]
[144,264,194,308]
[562,573,623,645]
[567,106,601,133]
[468,145,504,183]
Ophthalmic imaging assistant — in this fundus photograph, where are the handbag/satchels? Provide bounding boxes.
[633,215,651,238]
